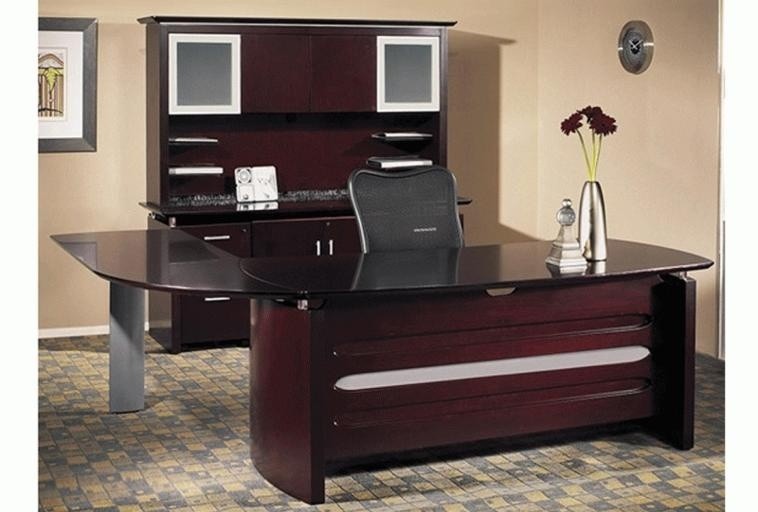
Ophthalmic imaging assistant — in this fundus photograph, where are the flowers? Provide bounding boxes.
[561,106,617,181]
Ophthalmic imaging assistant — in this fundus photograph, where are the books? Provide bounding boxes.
[367,156,433,169]
[169,137,220,143]
[370,131,432,138]
[168,167,224,175]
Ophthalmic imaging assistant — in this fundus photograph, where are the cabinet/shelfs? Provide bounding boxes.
[136,16,472,355]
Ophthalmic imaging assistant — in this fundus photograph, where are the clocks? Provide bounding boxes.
[617,20,654,75]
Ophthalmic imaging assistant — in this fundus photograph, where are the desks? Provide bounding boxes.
[50,227,714,504]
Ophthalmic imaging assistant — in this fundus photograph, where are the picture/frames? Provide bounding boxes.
[39,18,99,152]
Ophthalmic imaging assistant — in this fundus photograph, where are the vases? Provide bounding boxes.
[582,258,606,276]
[578,181,608,260]
